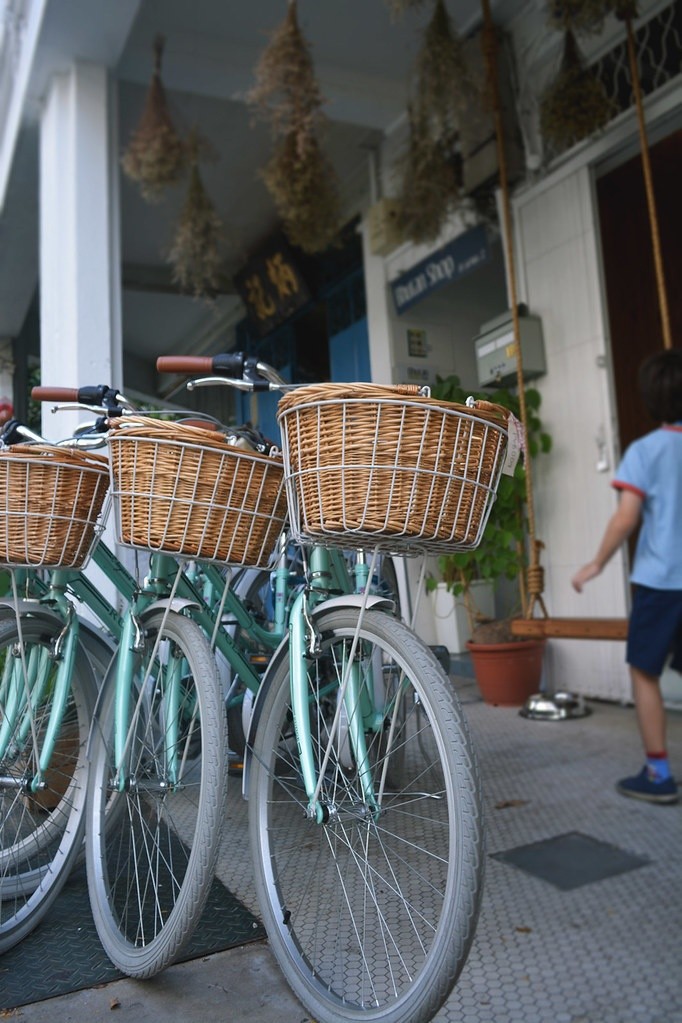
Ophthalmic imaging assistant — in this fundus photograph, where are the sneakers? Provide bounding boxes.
[616,765,679,802]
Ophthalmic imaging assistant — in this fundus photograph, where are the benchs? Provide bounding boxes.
[512,613,631,640]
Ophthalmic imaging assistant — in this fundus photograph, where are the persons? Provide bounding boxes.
[561,343,679,808]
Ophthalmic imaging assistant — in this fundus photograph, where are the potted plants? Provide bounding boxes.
[423,371,544,707]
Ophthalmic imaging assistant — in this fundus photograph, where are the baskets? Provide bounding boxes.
[106,413,294,571]
[274,381,514,559]
[0,443,109,572]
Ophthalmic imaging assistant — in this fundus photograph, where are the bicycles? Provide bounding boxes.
[0,351,526,1023]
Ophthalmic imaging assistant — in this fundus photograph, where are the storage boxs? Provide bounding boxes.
[276,381,521,553]
[109,415,292,568]
[0,444,112,567]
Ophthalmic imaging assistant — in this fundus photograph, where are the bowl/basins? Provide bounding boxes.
[518,691,587,723]
